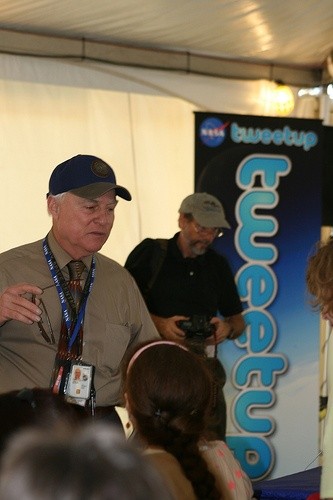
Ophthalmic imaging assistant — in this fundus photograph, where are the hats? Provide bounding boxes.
[179,193,231,229]
[49,155,133,202]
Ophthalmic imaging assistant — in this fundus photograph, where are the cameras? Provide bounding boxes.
[178,315,216,342]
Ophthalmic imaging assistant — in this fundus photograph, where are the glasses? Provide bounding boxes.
[32,294,55,346]
[192,225,224,238]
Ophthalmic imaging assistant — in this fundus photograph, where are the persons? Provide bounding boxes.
[0,153,254,500]
[307,235,333,329]
